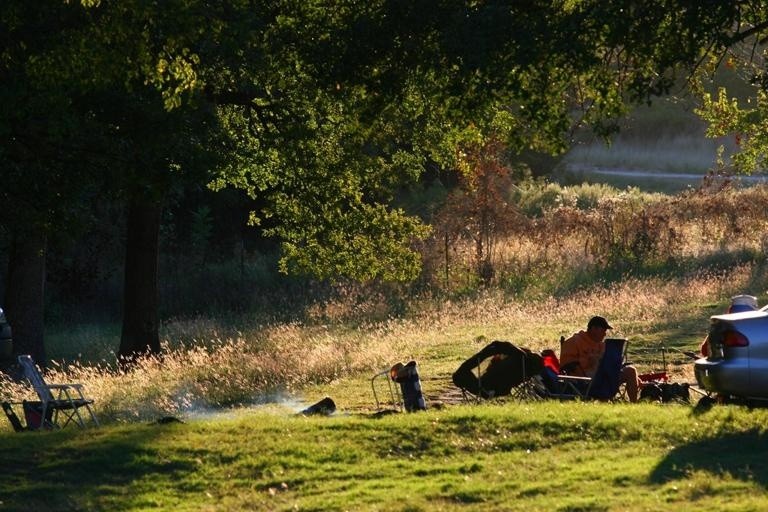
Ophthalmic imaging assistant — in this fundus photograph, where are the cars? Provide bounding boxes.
[693,303,768,402]
[0,308,12,342]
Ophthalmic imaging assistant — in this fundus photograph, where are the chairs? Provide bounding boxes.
[451,330,629,405]
[17,351,100,429]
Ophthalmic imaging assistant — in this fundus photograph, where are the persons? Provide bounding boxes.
[559,316,638,403]
[701,305,732,402]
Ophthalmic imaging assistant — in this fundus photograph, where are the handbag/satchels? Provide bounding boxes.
[659,381,689,403]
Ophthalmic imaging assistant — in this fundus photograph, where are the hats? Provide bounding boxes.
[588,316,612,329]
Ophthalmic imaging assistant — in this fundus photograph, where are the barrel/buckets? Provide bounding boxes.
[23,401,53,429]
[732,295,760,312]
